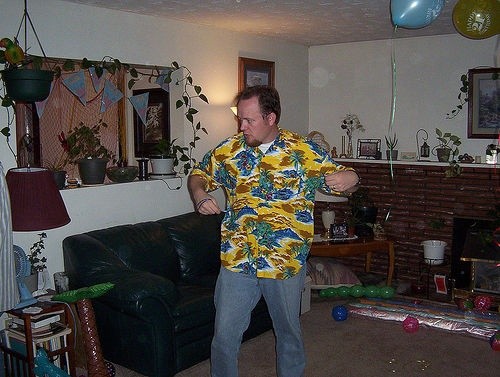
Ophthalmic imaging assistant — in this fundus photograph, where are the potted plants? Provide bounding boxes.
[0,38,200,188]
[421,208,453,265]
[431,128,462,177]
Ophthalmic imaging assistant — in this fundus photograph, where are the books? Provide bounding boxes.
[4,304,72,377]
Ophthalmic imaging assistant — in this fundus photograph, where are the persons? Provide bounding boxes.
[190,85,361,377]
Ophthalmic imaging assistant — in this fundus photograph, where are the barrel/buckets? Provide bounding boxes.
[420,240,448,264]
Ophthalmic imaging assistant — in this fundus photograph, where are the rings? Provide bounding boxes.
[333,185,336,189]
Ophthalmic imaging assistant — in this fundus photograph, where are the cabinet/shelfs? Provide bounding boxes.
[308,232,394,291]
[0,301,76,377]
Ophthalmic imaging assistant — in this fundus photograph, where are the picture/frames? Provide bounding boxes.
[468,68,500,139]
[357,139,381,159]
[131,88,170,157]
[237,57,276,131]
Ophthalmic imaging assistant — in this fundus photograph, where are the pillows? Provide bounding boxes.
[307,257,362,290]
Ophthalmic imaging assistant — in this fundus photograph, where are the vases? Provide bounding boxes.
[347,137,355,158]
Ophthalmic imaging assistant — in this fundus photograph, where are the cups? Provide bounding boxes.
[54,272,70,294]
[475,156,481,163]
[373,224,388,241]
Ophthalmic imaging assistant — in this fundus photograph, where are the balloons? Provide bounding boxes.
[332,305,346,321]
[402,317,419,333]
[459,299,473,311]
[320,285,396,300]
[490,331,500,351]
[475,295,491,312]
[452,0,500,40]
[390,0,445,29]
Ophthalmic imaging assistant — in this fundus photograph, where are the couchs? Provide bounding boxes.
[62,209,303,377]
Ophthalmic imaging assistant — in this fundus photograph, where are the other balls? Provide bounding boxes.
[319,285,395,299]
[475,295,492,314]
[332,305,348,321]
[489,330,500,351]
[402,317,419,334]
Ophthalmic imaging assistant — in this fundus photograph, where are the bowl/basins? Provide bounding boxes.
[107,166,138,183]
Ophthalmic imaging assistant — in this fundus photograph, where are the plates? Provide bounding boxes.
[459,160,474,163]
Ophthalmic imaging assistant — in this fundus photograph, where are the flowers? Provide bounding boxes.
[340,113,364,140]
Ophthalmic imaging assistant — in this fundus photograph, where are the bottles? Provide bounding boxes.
[486,144,500,165]
[421,142,430,157]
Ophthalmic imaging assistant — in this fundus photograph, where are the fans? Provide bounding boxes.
[12,244,37,311]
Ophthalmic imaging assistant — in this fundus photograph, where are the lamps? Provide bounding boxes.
[314,188,349,239]
[5,132,71,232]
